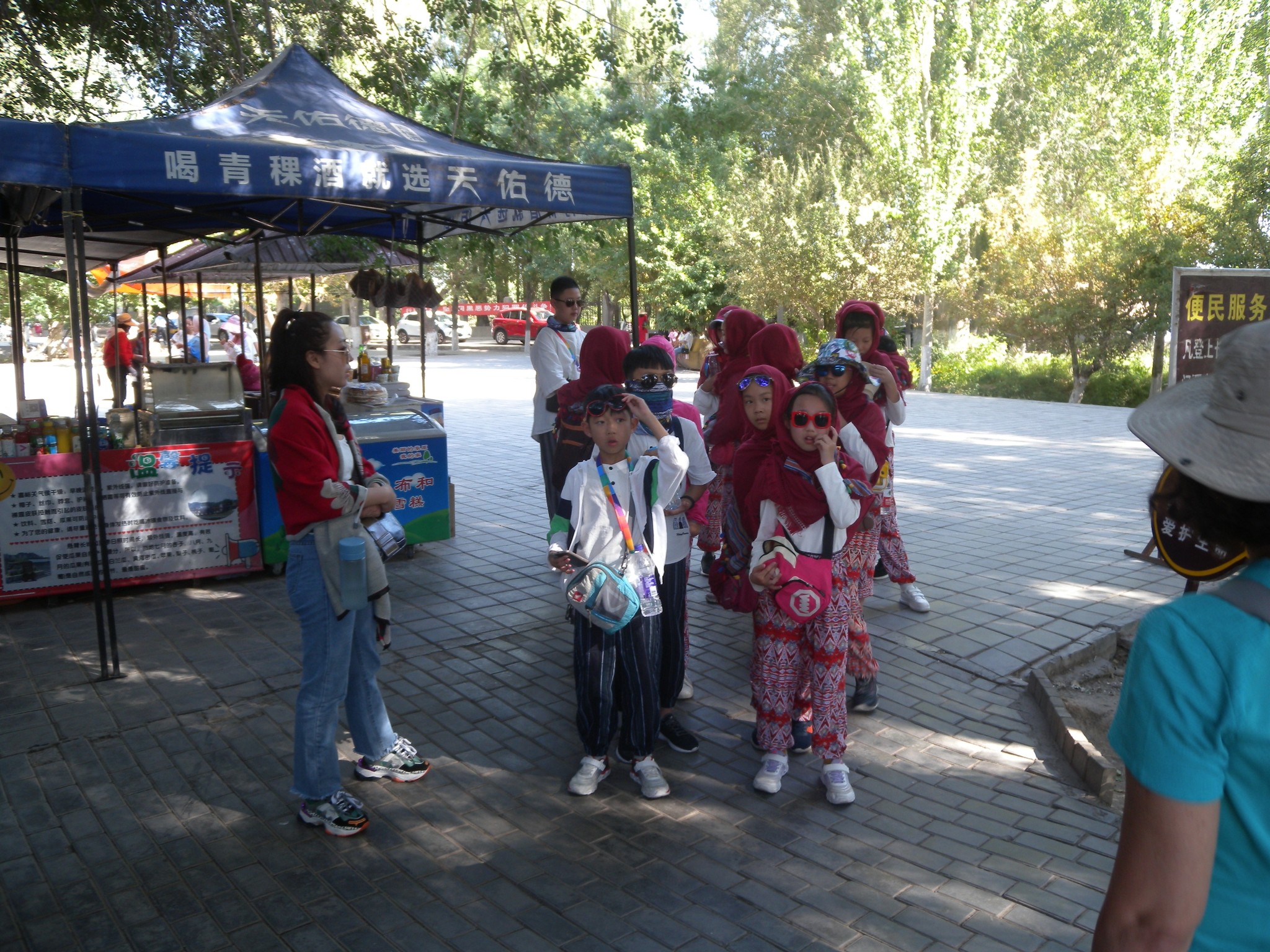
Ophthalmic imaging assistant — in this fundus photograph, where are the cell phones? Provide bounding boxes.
[552,550,589,567]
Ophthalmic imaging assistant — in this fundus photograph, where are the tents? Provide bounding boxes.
[10,37,642,678]
[0,113,108,679]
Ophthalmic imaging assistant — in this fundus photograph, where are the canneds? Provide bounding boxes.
[381,357,393,380]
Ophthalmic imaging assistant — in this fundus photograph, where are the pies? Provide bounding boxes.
[346,385,387,405]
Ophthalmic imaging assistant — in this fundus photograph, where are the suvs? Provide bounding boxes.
[491,307,581,345]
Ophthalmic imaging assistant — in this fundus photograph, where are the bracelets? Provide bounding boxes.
[680,496,695,510]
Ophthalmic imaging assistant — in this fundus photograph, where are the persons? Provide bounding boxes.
[657,327,693,367]
[102,312,133,405]
[591,344,717,763]
[260,303,428,835]
[528,271,589,572]
[128,322,156,389]
[551,326,631,504]
[639,334,709,699]
[1089,319,1270,951]
[219,315,259,365]
[170,314,211,363]
[692,299,931,804]
[546,384,690,798]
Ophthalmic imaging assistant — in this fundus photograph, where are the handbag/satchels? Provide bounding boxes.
[546,378,571,414]
[565,559,641,636]
[681,343,689,354]
[708,558,758,614]
[757,521,832,624]
[361,511,407,564]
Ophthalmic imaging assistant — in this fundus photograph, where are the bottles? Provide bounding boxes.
[108,413,124,449]
[634,544,663,617]
[14,424,31,458]
[648,447,682,511]
[360,344,371,383]
[0,426,16,458]
[70,420,82,453]
[56,419,71,454]
[12,424,18,437]
[42,421,58,454]
[357,344,363,383]
[28,422,44,456]
[708,350,721,378]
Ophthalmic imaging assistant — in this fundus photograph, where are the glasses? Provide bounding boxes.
[632,373,678,389]
[555,299,584,307]
[736,374,772,391]
[815,364,853,377]
[584,394,627,417]
[323,349,350,354]
[791,411,831,429]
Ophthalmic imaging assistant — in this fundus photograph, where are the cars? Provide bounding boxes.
[150,317,182,340]
[249,313,278,341]
[329,315,396,345]
[395,310,472,344]
[205,313,252,342]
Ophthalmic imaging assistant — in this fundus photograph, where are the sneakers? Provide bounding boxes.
[873,558,889,580]
[567,754,611,795]
[789,720,813,754]
[658,713,700,753]
[299,790,369,837]
[820,758,856,805]
[616,728,633,764]
[852,674,880,712]
[354,735,431,782]
[753,751,789,793]
[900,586,930,612]
[751,727,767,750]
[630,755,670,798]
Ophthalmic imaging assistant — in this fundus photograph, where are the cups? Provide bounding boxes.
[388,373,399,382]
[348,370,354,382]
[337,488,368,611]
[389,365,400,373]
[378,374,388,383]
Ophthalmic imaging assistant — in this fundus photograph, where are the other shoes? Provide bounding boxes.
[678,670,694,699]
[700,551,716,576]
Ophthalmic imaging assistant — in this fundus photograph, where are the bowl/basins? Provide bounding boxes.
[370,365,382,381]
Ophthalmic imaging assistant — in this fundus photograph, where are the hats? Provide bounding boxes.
[796,338,881,387]
[117,312,137,326]
[220,315,246,333]
[709,319,723,330]
[137,322,144,333]
[1127,316,1270,501]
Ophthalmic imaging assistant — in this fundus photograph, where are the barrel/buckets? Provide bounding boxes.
[105,407,136,448]
